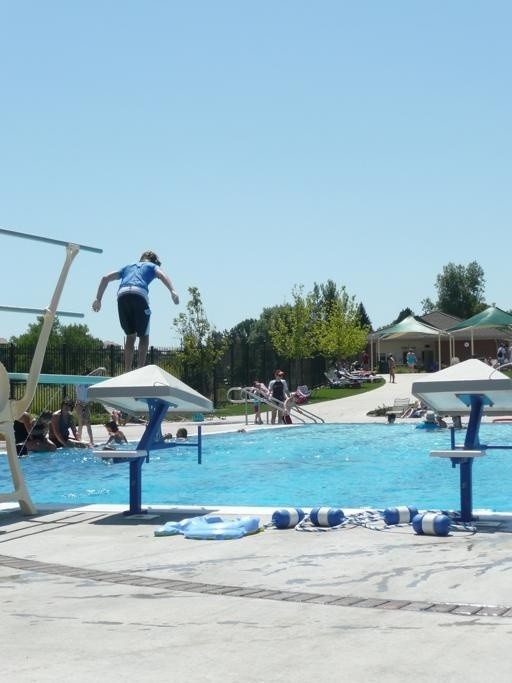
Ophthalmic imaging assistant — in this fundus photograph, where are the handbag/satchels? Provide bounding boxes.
[497,350,502,357]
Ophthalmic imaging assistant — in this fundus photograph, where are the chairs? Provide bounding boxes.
[385,398,410,417]
[324,366,382,389]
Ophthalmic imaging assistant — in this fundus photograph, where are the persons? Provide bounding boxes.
[296,385,311,403]
[253,370,291,424]
[14,366,106,458]
[415,411,446,430]
[165,429,187,443]
[387,413,396,424]
[407,349,417,372]
[482,343,511,366]
[100,421,128,446]
[334,350,374,384]
[92,252,179,373]
[388,353,396,384]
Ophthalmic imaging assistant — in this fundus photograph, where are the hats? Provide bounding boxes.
[423,411,437,422]
